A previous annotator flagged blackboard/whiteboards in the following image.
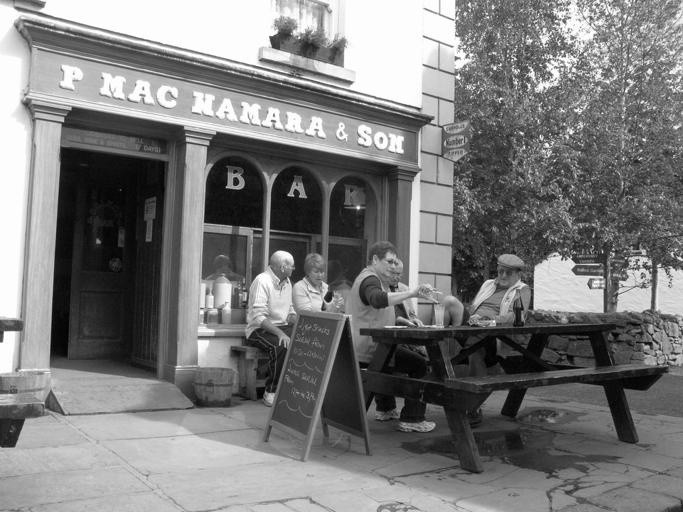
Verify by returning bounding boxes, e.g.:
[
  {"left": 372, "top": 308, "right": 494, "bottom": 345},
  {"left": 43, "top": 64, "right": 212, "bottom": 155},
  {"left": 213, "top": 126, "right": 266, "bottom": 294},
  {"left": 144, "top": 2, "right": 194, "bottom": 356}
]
[{"left": 268, "top": 310, "right": 347, "bottom": 443}]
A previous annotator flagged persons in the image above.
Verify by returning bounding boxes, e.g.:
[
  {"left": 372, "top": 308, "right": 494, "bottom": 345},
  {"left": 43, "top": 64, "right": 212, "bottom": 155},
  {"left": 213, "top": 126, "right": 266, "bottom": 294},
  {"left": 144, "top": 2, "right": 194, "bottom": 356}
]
[
  {"left": 293, "top": 253, "right": 345, "bottom": 314},
  {"left": 245, "top": 250, "right": 297, "bottom": 407},
  {"left": 205, "top": 255, "right": 244, "bottom": 281},
  {"left": 441, "top": 254, "right": 532, "bottom": 428},
  {"left": 344, "top": 241, "right": 442, "bottom": 432}
]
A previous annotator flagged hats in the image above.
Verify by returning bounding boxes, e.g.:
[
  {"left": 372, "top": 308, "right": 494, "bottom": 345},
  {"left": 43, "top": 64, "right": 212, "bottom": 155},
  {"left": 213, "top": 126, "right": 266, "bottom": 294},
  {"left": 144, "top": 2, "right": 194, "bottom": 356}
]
[{"left": 498, "top": 254, "right": 525, "bottom": 271}]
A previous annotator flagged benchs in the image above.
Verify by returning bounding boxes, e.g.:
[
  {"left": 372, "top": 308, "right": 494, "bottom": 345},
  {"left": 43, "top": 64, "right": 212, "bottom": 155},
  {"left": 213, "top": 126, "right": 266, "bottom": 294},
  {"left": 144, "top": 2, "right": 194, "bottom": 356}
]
[{"left": 232, "top": 344, "right": 268, "bottom": 401}]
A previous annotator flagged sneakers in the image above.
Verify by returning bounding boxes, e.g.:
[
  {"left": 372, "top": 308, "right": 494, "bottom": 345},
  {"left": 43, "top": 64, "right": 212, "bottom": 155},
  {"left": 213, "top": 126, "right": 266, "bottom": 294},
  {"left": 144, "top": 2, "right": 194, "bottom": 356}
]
[
  {"left": 374, "top": 409, "right": 400, "bottom": 421},
  {"left": 468, "top": 410, "right": 483, "bottom": 428},
  {"left": 263, "top": 391, "right": 276, "bottom": 407},
  {"left": 396, "top": 420, "right": 436, "bottom": 433}
]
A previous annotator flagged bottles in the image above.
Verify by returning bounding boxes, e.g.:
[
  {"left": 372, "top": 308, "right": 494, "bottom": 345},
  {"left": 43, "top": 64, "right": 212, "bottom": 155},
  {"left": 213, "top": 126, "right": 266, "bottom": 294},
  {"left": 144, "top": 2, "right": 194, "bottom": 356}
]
[
  {"left": 222, "top": 300, "right": 231, "bottom": 326},
  {"left": 206, "top": 287, "right": 215, "bottom": 309},
  {"left": 206, "top": 309, "right": 218, "bottom": 327},
  {"left": 199, "top": 307, "right": 204, "bottom": 325},
  {"left": 214, "top": 272, "right": 232, "bottom": 308},
  {"left": 235, "top": 278, "right": 248, "bottom": 308},
  {"left": 512, "top": 288, "right": 526, "bottom": 328}
]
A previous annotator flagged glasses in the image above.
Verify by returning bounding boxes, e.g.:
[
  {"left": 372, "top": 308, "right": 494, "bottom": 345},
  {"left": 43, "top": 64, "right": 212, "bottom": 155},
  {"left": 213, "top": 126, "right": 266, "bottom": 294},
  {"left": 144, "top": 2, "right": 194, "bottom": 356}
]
[
  {"left": 384, "top": 259, "right": 399, "bottom": 266},
  {"left": 498, "top": 269, "right": 513, "bottom": 276}
]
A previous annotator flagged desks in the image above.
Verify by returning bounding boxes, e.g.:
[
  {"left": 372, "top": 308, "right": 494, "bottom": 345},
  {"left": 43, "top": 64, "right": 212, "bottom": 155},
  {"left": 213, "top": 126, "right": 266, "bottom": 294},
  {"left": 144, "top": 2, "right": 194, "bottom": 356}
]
[{"left": 358, "top": 320, "right": 641, "bottom": 471}]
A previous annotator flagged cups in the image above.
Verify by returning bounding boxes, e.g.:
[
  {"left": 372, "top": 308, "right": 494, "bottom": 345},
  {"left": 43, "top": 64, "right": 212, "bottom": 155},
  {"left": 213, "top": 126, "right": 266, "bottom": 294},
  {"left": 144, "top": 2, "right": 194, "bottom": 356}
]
[
  {"left": 433, "top": 303, "right": 445, "bottom": 327},
  {"left": 418, "top": 289, "right": 443, "bottom": 303}
]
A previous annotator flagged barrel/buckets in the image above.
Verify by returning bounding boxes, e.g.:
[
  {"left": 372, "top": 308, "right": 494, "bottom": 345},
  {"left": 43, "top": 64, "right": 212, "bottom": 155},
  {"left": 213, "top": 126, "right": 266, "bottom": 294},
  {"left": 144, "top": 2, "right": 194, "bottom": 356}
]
[
  {"left": 192, "top": 366, "right": 238, "bottom": 407},
  {"left": 0, "top": 371, "right": 53, "bottom": 408}
]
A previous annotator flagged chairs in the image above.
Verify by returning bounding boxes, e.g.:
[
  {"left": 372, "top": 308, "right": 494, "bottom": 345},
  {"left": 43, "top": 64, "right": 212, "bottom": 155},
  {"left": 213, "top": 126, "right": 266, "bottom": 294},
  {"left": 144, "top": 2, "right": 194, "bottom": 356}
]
[{"left": 441, "top": 353, "right": 669, "bottom": 473}]
